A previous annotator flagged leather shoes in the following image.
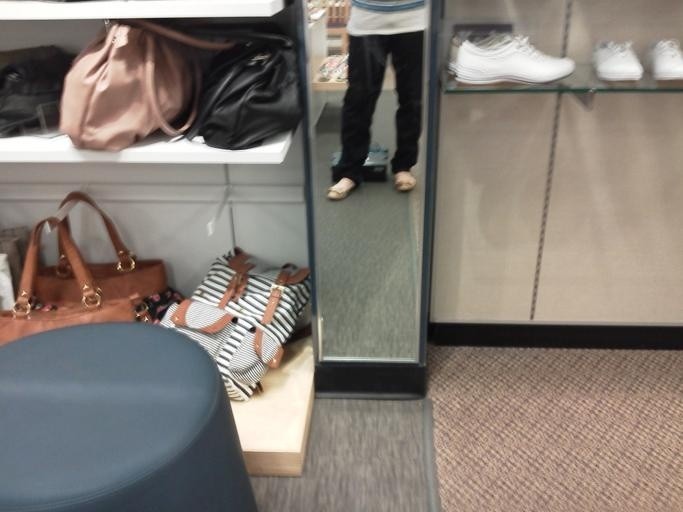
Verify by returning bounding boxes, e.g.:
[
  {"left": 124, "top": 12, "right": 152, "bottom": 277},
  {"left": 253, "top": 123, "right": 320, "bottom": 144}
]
[
  {"left": 328, "top": 186, "right": 355, "bottom": 200},
  {"left": 394, "top": 171, "right": 415, "bottom": 192}
]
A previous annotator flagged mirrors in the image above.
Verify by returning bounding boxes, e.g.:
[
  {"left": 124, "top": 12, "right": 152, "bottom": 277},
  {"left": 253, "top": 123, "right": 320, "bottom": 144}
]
[{"left": 297, "top": 0, "right": 440, "bottom": 400}]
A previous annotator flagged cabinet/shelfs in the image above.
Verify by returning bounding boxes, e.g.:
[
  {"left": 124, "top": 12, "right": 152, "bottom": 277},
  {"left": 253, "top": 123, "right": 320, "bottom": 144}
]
[
  {"left": 430, "top": 2, "right": 683, "bottom": 349},
  {"left": 0, "top": 1, "right": 313, "bottom": 476}
]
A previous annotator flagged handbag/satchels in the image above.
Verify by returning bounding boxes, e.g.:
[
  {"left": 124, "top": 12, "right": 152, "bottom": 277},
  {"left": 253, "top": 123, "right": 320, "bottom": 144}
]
[
  {"left": 1, "top": 46, "right": 79, "bottom": 138},
  {"left": 159, "top": 276, "right": 285, "bottom": 401},
  {"left": 0, "top": 217, "right": 151, "bottom": 346},
  {"left": 186, "top": 21, "right": 303, "bottom": 150},
  {"left": 139, "top": 288, "right": 185, "bottom": 325},
  {"left": 1, "top": 254, "right": 16, "bottom": 311},
  {"left": 191, "top": 246, "right": 314, "bottom": 346},
  {"left": 0, "top": 225, "right": 31, "bottom": 294},
  {"left": 58, "top": 18, "right": 237, "bottom": 153},
  {"left": 35, "top": 191, "right": 166, "bottom": 297}
]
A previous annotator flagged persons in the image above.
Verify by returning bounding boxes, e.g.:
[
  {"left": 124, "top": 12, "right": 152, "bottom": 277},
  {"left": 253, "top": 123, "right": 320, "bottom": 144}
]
[{"left": 325, "top": 0, "right": 429, "bottom": 200}]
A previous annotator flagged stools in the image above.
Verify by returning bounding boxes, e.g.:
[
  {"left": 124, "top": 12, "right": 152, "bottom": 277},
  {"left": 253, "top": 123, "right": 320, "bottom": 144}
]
[{"left": 1, "top": 321, "right": 261, "bottom": 511}]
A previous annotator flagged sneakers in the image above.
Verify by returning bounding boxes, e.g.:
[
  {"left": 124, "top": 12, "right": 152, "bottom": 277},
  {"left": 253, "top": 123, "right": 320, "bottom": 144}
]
[
  {"left": 591, "top": 40, "right": 644, "bottom": 82},
  {"left": 457, "top": 41, "right": 574, "bottom": 83},
  {"left": 447, "top": 31, "right": 514, "bottom": 73},
  {"left": 643, "top": 39, "right": 682, "bottom": 80}
]
